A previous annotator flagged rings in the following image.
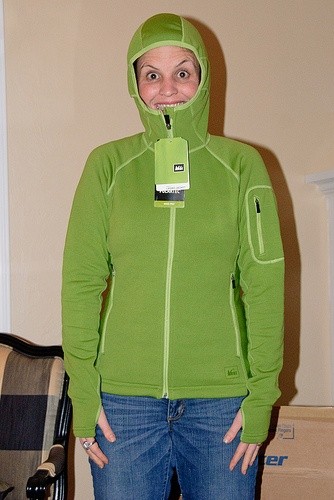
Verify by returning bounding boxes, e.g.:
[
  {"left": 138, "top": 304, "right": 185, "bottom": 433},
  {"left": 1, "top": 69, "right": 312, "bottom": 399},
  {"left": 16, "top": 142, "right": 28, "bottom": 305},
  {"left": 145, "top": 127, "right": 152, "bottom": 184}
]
[{"left": 83, "top": 441, "right": 97, "bottom": 450}]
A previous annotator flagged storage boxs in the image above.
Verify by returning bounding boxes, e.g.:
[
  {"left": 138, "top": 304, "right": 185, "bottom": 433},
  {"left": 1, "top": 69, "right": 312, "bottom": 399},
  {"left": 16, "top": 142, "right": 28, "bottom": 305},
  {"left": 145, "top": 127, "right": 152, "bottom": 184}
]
[{"left": 257, "top": 406, "right": 334, "bottom": 500}]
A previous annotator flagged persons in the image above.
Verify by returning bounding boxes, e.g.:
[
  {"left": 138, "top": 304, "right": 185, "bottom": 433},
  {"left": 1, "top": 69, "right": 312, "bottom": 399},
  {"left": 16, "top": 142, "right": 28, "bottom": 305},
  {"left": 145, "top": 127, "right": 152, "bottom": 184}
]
[{"left": 61, "top": 12, "right": 286, "bottom": 500}]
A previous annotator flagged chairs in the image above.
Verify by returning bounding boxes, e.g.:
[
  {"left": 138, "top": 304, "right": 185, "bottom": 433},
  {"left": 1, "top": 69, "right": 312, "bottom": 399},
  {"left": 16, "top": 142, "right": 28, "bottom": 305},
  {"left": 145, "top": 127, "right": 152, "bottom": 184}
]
[{"left": 0, "top": 333, "right": 76, "bottom": 500}]
